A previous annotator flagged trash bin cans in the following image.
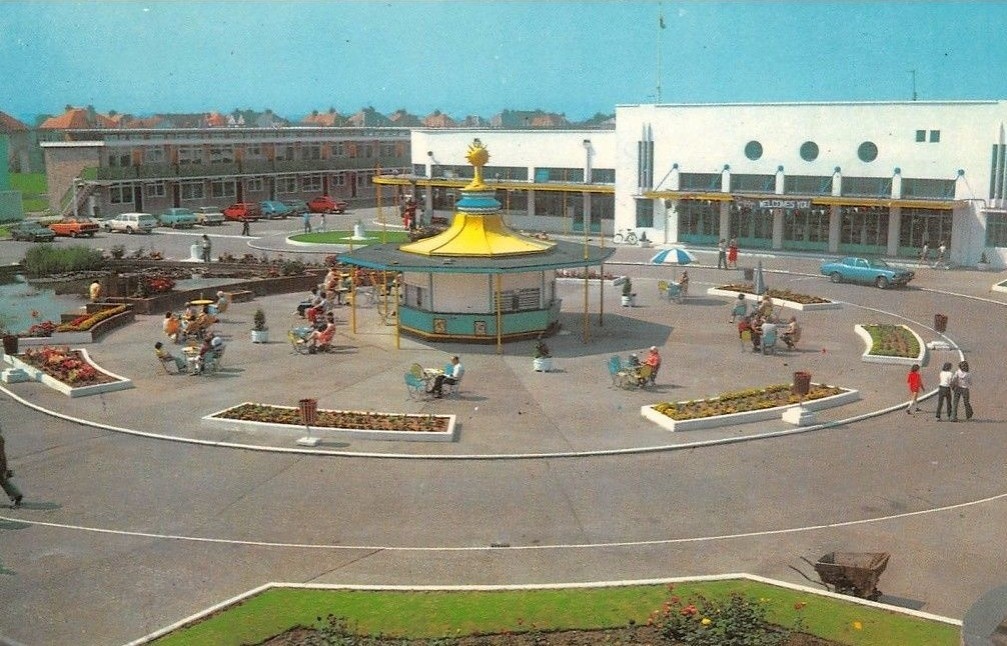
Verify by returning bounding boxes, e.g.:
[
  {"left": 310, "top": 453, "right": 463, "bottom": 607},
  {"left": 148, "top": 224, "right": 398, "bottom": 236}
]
[
  {"left": 933, "top": 313, "right": 947, "bottom": 333},
  {"left": 299, "top": 399, "right": 317, "bottom": 426},
  {"left": 792, "top": 371, "right": 811, "bottom": 396},
  {"left": 744, "top": 267, "right": 754, "bottom": 281},
  {"left": 3, "top": 335, "right": 18, "bottom": 354}
]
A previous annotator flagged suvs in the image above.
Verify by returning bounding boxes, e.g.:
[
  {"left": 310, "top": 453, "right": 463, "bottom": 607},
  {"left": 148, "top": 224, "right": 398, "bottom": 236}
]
[
  {"left": 104, "top": 213, "right": 159, "bottom": 234},
  {"left": 225, "top": 202, "right": 262, "bottom": 222}
]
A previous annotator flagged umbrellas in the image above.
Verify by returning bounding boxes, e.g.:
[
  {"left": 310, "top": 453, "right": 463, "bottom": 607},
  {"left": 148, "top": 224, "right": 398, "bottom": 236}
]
[
  {"left": 754, "top": 263, "right": 766, "bottom": 302},
  {"left": 650, "top": 247, "right": 697, "bottom": 282}
]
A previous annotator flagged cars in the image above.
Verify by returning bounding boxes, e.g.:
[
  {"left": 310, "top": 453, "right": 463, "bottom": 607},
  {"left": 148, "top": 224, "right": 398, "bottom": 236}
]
[
  {"left": 284, "top": 200, "right": 310, "bottom": 217},
  {"left": 820, "top": 255, "right": 916, "bottom": 289},
  {"left": 306, "top": 195, "right": 348, "bottom": 214},
  {"left": 259, "top": 201, "right": 288, "bottom": 220},
  {"left": 193, "top": 206, "right": 226, "bottom": 226},
  {"left": 11, "top": 223, "right": 56, "bottom": 244},
  {"left": 49, "top": 216, "right": 100, "bottom": 238},
  {"left": 157, "top": 207, "right": 199, "bottom": 228}
]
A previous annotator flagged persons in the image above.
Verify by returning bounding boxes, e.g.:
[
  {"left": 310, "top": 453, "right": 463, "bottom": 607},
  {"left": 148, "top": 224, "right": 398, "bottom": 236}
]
[
  {"left": 88, "top": 279, "right": 101, "bottom": 303},
  {"left": 730, "top": 293, "right": 799, "bottom": 350},
  {"left": 642, "top": 345, "right": 661, "bottom": 371},
  {"left": 918, "top": 241, "right": 949, "bottom": 270},
  {"left": 950, "top": 361, "right": 974, "bottom": 421},
  {"left": 678, "top": 270, "right": 689, "bottom": 284},
  {"left": 718, "top": 239, "right": 738, "bottom": 270},
  {"left": 935, "top": 362, "right": 953, "bottom": 421},
  {"left": 906, "top": 364, "right": 925, "bottom": 414},
  {"left": 155, "top": 267, "right": 402, "bottom": 376},
  {"left": 303, "top": 211, "right": 312, "bottom": 234},
  {"left": 0, "top": 436, "right": 23, "bottom": 505},
  {"left": 426, "top": 355, "right": 464, "bottom": 397},
  {"left": 201, "top": 235, "right": 211, "bottom": 262}
]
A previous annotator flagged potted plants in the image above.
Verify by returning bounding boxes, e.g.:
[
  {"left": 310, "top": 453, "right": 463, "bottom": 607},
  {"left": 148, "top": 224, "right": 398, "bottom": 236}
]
[
  {"left": 251, "top": 310, "right": 269, "bottom": 343},
  {"left": 622, "top": 279, "right": 636, "bottom": 307},
  {"left": 534, "top": 338, "right": 553, "bottom": 372}
]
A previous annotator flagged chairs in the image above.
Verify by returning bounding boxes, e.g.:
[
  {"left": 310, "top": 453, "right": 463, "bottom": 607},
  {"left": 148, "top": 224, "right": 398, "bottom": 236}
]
[
  {"left": 608, "top": 355, "right": 651, "bottom": 391},
  {"left": 733, "top": 303, "right": 802, "bottom": 355},
  {"left": 404, "top": 363, "right": 462, "bottom": 402},
  {"left": 160, "top": 343, "right": 226, "bottom": 377},
  {"left": 658, "top": 280, "right": 688, "bottom": 304}
]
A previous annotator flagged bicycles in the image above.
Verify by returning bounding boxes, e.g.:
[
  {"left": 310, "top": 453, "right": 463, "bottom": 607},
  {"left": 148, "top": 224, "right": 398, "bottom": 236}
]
[{"left": 612, "top": 229, "right": 641, "bottom": 245}]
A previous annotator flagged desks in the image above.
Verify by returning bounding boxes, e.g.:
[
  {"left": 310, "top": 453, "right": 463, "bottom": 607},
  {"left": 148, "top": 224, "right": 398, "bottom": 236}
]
[{"left": 191, "top": 299, "right": 214, "bottom": 312}]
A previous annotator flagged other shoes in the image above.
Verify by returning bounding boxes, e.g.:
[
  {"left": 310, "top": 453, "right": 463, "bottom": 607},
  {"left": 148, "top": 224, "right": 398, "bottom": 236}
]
[
  {"left": 915, "top": 408, "right": 922, "bottom": 411},
  {"left": 426, "top": 390, "right": 434, "bottom": 393},
  {"left": 936, "top": 415, "right": 940, "bottom": 419},
  {"left": 968, "top": 411, "right": 973, "bottom": 419},
  {"left": 435, "top": 395, "right": 442, "bottom": 398},
  {"left": 906, "top": 410, "right": 911, "bottom": 414},
  {"left": 16, "top": 496, "right": 22, "bottom": 506}
]
